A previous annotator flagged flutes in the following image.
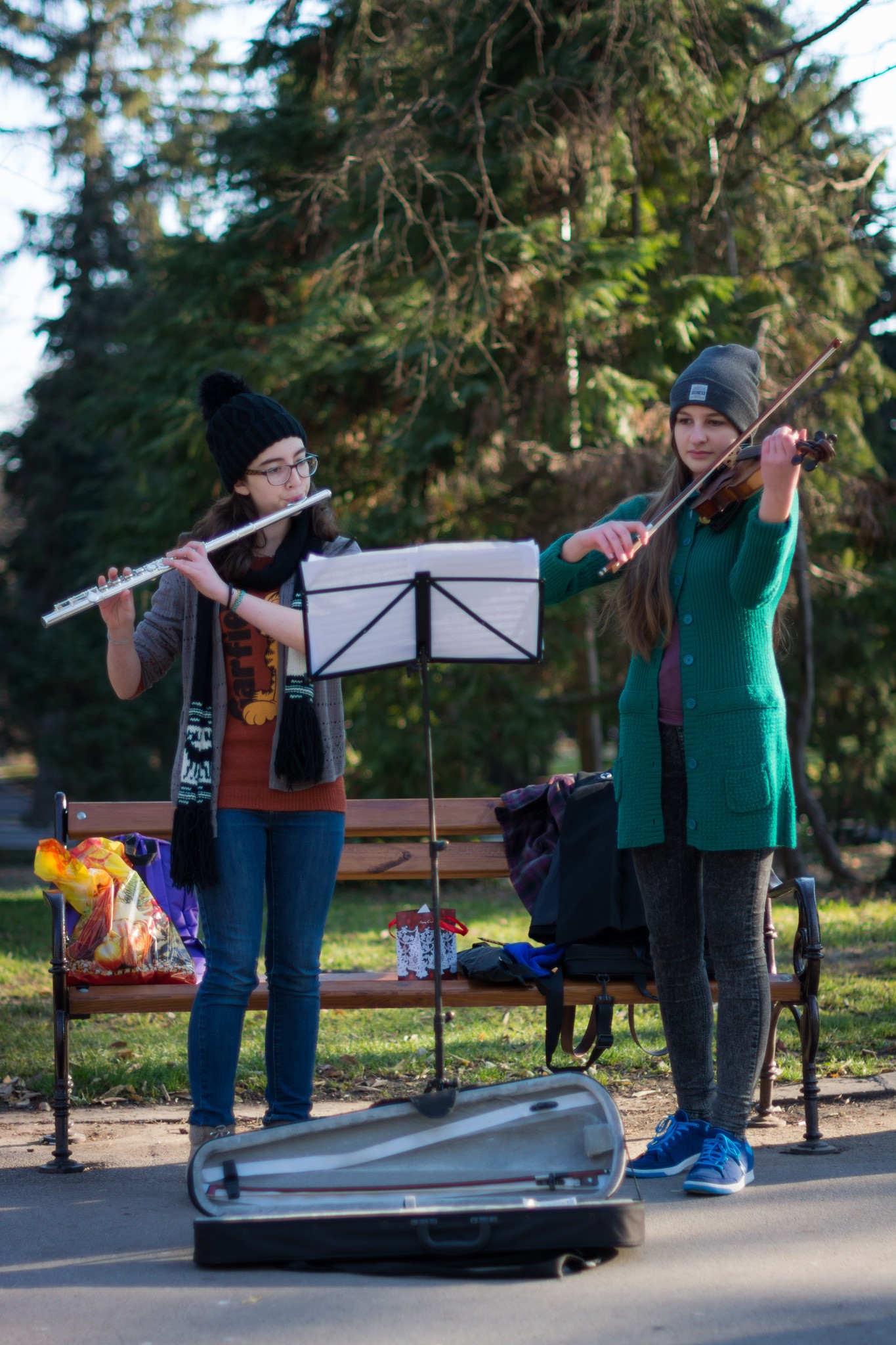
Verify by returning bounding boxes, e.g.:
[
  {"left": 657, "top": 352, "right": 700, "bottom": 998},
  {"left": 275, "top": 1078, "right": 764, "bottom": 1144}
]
[{"left": 38, "top": 488, "right": 332, "bottom": 629}]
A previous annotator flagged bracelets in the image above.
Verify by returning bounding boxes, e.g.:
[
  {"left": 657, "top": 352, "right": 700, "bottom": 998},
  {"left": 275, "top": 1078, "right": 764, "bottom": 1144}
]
[{"left": 224, "top": 582, "right": 246, "bottom": 613}]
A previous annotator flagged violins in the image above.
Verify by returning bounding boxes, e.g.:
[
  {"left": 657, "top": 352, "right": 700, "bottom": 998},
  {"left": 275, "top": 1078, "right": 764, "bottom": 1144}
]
[{"left": 683, "top": 430, "right": 840, "bottom": 524}]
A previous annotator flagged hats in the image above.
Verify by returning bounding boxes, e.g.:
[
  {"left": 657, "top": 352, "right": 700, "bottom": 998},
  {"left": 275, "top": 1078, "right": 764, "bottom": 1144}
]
[
  {"left": 669, "top": 343, "right": 761, "bottom": 441},
  {"left": 196, "top": 369, "right": 307, "bottom": 496}
]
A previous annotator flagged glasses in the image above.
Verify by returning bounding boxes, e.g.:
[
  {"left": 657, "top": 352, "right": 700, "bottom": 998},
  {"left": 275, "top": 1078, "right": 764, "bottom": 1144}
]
[{"left": 245, "top": 452, "right": 319, "bottom": 486}]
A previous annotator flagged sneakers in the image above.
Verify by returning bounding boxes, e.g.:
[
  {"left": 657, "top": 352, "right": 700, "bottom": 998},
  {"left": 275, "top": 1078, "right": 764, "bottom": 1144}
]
[
  {"left": 185, "top": 1124, "right": 235, "bottom": 1183},
  {"left": 683, "top": 1128, "right": 755, "bottom": 1195},
  {"left": 625, "top": 1109, "right": 710, "bottom": 1178}
]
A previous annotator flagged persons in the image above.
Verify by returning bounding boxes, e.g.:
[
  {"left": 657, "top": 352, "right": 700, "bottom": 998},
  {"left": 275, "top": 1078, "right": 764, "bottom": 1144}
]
[
  {"left": 539, "top": 343, "right": 810, "bottom": 1194},
  {"left": 96, "top": 372, "right": 361, "bottom": 1161}
]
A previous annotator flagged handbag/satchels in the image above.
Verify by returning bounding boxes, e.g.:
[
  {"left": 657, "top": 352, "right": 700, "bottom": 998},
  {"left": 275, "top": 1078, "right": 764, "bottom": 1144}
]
[
  {"left": 34, "top": 836, "right": 198, "bottom": 986},
  {"left": 388, "top": 908, "right": 469, "bottom": 981}
]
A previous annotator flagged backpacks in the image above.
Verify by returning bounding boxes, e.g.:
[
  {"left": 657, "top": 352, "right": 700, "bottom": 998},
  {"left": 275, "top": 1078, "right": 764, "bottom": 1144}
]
[
  {"left": 527, "top": 769, "right": 656, "bottom": 985},
  {"left": 65, "top": 833, "right": 207, "bottom": 976}
]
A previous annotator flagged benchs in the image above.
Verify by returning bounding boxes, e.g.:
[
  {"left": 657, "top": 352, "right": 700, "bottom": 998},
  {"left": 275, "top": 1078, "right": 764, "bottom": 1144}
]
[{"left": 39, "top": 790, "right": 840, "bottom": 1172}]
